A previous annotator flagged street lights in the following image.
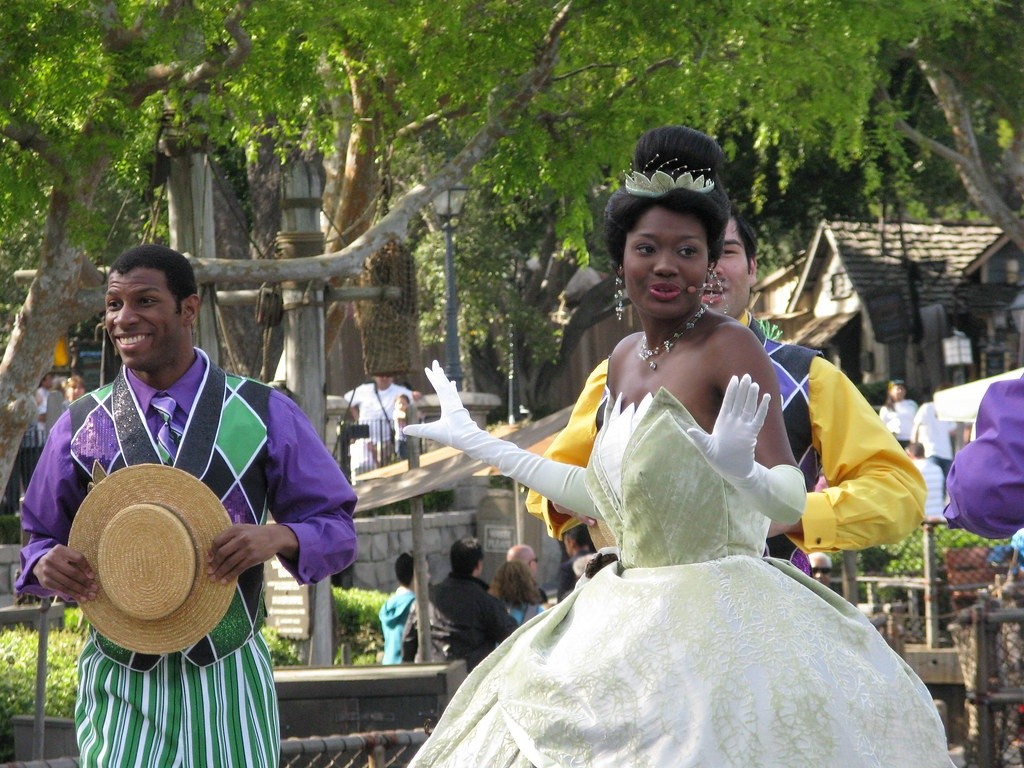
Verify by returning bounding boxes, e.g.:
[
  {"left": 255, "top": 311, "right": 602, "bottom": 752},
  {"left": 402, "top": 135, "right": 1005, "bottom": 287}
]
[
  {"left": 499, "top": 245, "right": 526, "bottom": 425},
  {"left": 433, "top": 181, "right": 469, "bottom": 391},
  {"left": 337, "top": 422, "right": 371, "bottom": 592}
]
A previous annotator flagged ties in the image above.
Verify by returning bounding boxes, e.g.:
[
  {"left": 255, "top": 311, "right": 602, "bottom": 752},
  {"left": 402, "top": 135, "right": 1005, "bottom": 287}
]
[{"left": 150, "top": 395, "right": 183, "bottom": 466}]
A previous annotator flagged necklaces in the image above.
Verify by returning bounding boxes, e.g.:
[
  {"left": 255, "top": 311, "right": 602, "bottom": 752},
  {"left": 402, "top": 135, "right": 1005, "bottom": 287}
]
[{"left": 639, "top": 303, "right": 710, "bottom": 371}]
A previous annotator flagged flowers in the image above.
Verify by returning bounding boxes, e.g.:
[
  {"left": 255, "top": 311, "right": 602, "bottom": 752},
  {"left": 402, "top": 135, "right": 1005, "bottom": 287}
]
[{"left": 621, "top": 160, "right": 716, "bottom": 196}]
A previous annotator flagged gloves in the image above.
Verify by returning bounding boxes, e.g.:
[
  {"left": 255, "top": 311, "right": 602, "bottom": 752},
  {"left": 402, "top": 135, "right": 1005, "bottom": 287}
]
[
  {"left": 687, "top": 370, "right": 809, "bottom": 538},
  {"left": 401, "top": 361, "right": 600, "bottom": 528}
]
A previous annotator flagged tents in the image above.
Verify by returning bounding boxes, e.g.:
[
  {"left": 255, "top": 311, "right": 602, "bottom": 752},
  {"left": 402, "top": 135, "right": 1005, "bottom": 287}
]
[{"left": 932, "top": 368, "right": 1024, "bottom": 443}]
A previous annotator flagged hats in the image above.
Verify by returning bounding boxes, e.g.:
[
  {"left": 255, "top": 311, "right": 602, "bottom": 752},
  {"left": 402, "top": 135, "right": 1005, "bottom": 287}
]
[{"left": 67, "top": 464, "right": 237, "bottom": 657}]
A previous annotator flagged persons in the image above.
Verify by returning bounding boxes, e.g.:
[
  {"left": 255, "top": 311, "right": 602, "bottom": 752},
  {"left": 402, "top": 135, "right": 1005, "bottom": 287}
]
[
  {"left": 378, "top": 524, "right": 598, "bottom": 665},
  {"left": 10, "top": 244, "right": 360, "bottom": 768},
  {"left": 524, "top": 202, "right": 927, "bottom": 577},
  {"left": 2, "top": 372, "right": 86, "bottom": 515},
  {"left": 878, "top": 367, "right": 1024, "bottom": 600},
  {"left": 338, "top": 364, "right": 423, "bottom": 484},
  {"left": 807, "top": 467, "right": 833, "bottom": 592},
  {"left": 402, "top": 123, "right": 951, "bottom": 768}
]
